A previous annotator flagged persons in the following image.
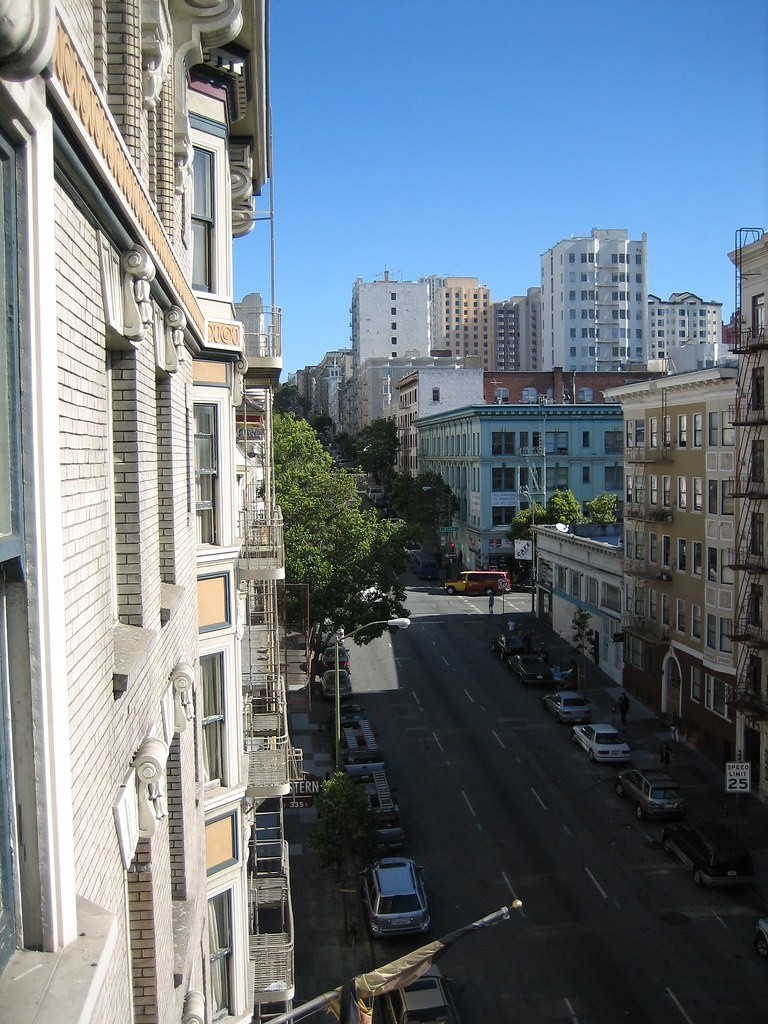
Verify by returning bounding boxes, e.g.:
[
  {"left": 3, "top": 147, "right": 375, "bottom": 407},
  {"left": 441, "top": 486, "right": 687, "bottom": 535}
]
[
  {"left": 489, "top": 593, "right": 494, "bottom": 615},
  {"left": 618, "top": 693, "right": 630, "bottom": 726},
  {"left": 507, "top": 617, "right": 578, "bottom": 678},
  {"left": 659, "top": 743, "right": 671, "bottom": 774},
  {"left": 670, "top": 711, "right": 682, "bottom": 744}
]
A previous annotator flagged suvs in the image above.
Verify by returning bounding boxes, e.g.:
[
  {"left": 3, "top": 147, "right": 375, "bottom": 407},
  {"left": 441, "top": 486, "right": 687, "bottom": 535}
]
[
  {"left": 663, "top": 822, "right": 754, "bottom": 889},
  {"left": 359, "top": 856, "right": 430, "bottom": 940},
  {"left": 615, "top": 769, "right": 688, "bottom": 822}
]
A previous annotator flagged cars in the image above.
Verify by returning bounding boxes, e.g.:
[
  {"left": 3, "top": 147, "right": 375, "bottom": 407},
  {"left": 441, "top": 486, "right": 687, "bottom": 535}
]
[
  {"left": 571, "top": 723, "right": 631, "bottom": 765},
  {"left": 318, "top": 669, "right": 353, "bottom": 701},
  {"left": 505, "top": 655, "right": 554, "bottom": 686},
  {"left": 542, "top": 691, "right": 592, "bottom": 724},
  {"left": 380, "top": 963, "right": 462, "bottom": 1024},
  {"left": 753, "top": 918, "right": 768, "bottom": 959},
  {"left": 329, "top": 704, "right": 365, "bottom": 730},
  {"left": 492, "top": 633, "right": 526, "bottom": 661},
  {"left": 320, "top": 630, "right": 351, "bottom": 676},
  {"left": 317, "top": 432, "right": 399, "bottom": 523},
  {"left": 416, "top": 558, "right": 440, "bottom": 581}
]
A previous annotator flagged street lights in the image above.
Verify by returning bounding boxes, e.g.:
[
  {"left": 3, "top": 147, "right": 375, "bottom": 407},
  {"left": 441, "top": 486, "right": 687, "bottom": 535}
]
[
  {"left": 335, "top": 618, "right": 411, "bottom": 775},
  {"left": 422, "top": 486, "right": 451, "bottom": 580}
]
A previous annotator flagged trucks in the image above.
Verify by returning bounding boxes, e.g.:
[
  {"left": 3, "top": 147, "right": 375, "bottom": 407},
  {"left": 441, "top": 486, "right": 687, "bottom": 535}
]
[{"left": 337, "top": 720, "right": 406, "bottom": 853}]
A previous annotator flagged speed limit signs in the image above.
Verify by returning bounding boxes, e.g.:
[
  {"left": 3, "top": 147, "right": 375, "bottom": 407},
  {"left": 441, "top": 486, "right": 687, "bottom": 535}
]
[{"left": 725, "top": 761, "right": 752, "bottom": 795}]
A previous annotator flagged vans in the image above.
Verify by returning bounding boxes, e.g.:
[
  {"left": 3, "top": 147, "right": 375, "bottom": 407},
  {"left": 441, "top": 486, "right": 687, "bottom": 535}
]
[{"left": 444, "top": 570, "right": 512, "bottom": 595}]
